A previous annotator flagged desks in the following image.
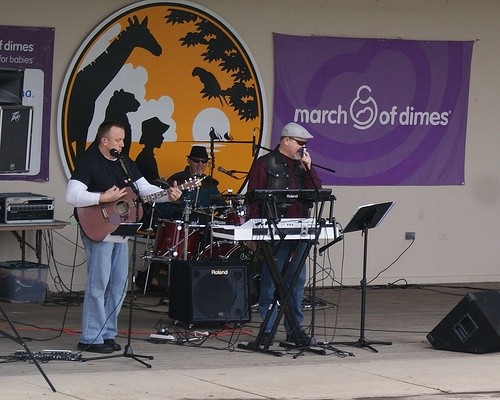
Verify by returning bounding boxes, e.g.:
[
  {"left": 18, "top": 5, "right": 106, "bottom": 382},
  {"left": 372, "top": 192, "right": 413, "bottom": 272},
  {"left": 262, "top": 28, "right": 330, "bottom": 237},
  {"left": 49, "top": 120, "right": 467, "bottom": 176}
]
[{"left": 0, "top": 220, "right": 71, "bottom": 282}]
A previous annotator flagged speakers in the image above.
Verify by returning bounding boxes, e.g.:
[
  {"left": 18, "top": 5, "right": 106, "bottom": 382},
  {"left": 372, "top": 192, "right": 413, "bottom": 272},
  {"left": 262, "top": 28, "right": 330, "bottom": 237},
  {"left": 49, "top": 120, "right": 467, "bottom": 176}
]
[
  {"left": 0, "top": 69, "right": 34, "bottom": 174},
  {"left": 169, "top": 260, "right": 251, "bottom": 324},
  {"left": 425, "top": 291, "right": 500, "bottom": 354}
]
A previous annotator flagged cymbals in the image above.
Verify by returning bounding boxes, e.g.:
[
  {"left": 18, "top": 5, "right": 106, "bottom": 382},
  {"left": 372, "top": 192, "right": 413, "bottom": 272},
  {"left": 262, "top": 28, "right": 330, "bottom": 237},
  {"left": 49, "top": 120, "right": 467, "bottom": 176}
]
[
  {"left": 188, "top": 205, "right": 232, "bottom": 220},
  {"left": 207, "top": 193, "right": 247, "bottom": 200}
]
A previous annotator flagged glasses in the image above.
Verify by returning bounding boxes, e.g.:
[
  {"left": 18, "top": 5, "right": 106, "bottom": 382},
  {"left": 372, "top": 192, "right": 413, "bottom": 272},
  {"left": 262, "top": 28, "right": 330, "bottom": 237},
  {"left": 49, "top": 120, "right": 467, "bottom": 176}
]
[
  {"left": 193, "top": 159, "right": 208, "bottom": 164},
  {"left": 289, "top": 136, "right": 308, "bottom": 146}
]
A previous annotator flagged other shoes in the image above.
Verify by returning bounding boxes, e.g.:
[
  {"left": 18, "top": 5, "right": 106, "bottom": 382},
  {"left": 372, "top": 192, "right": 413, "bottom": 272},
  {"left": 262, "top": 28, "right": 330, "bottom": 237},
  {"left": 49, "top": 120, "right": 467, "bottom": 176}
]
[
  {"left": 286, "top": 332, "right": 315, "bottom": 344},
  {"left": 102, "top": 339, "right": 121, "bottom": 351},
  {"left": 257, "top": 332, "right": 275, "bottom": 346},
  {"left": 77, "top": 341, "right": 114, "bottom": 353}
]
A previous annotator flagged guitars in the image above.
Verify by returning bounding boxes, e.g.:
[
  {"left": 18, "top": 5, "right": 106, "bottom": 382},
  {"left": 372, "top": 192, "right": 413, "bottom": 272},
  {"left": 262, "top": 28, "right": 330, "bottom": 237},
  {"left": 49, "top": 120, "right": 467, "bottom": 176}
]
[{"left": 73, "top": 176, "right": 204, "bottom": 242}]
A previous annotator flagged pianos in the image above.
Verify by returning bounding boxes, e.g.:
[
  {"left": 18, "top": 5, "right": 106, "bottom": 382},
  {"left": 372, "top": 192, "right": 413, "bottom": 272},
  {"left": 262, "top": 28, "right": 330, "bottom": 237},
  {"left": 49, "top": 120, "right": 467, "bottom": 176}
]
[{"left": 208, "top": 217, "right": 339, "bottom": 357}]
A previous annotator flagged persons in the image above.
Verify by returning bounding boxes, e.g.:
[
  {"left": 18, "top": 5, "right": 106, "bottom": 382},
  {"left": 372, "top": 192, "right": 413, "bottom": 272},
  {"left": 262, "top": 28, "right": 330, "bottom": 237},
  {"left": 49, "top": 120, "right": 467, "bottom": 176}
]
[
  {"left": 64, "top": 121, "right": 183, "bottom": 354},
  {"left": 240, "top": 123, "right": 324, "bottom": 347},
  {"left": 161, "top": 146, "right": 232, "bottom": 220}
]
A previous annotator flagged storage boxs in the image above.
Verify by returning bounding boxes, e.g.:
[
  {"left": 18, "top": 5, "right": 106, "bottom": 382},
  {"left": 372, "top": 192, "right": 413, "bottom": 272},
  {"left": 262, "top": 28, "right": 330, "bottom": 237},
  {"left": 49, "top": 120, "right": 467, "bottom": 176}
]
[
  {"left": 0, "top": 261, "right": 49, "bottom": 304},
  {"left": 0, "top": 192, "right": 55, "bottom": 223}
]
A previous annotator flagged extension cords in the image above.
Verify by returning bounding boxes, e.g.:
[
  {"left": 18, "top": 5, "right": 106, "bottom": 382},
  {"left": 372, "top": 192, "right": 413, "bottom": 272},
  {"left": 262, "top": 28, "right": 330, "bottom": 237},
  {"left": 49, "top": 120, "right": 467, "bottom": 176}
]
[{"left": 149, "top": 333, "right": 175, "bottom": 339}]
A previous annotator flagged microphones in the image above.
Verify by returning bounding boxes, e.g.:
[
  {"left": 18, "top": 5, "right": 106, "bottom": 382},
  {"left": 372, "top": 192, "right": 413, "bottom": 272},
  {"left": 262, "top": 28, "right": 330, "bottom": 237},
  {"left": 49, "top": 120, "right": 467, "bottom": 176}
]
[
  {"left": 217, "top": 166, "right": 239, "bottom": 179},
  {"left": 252, "top": 128, "right": 255, "bottom": 156},
  {"left": 110, "top": 149, "right": 126, "bottom": 160}
]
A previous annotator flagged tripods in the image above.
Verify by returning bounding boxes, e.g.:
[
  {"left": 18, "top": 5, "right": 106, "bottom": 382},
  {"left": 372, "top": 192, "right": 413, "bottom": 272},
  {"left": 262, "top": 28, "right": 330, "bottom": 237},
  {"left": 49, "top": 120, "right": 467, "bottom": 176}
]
[
  {"left": 81, "top": 159, "right": 154, "bottom": 367},
  {"left": 288, "top": 201, "right": 396, "bottom": 359}
]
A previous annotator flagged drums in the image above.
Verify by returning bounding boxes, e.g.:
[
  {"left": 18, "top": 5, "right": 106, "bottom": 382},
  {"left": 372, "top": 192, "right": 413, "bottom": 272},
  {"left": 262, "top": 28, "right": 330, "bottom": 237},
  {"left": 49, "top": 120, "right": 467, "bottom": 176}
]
[
  {"left": 224, "top": 205, "right": 247, "bottom": 226},
  {"left": 152, "top": 218, "right": 205, "bottom": 262},
  {"left": 195, "top": 240, "right": 263, "bottom": 308}
]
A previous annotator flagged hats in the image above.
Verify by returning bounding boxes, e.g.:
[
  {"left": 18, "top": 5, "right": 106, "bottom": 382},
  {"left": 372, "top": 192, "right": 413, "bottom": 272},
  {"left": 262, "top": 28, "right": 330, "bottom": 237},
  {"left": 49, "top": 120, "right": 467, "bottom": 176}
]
[
  {"left": 186, "top": 145, "right": 213, "bottom": 161},
  {"left": 280, "top": 122, "right": 314, "bottom": 139}
]
